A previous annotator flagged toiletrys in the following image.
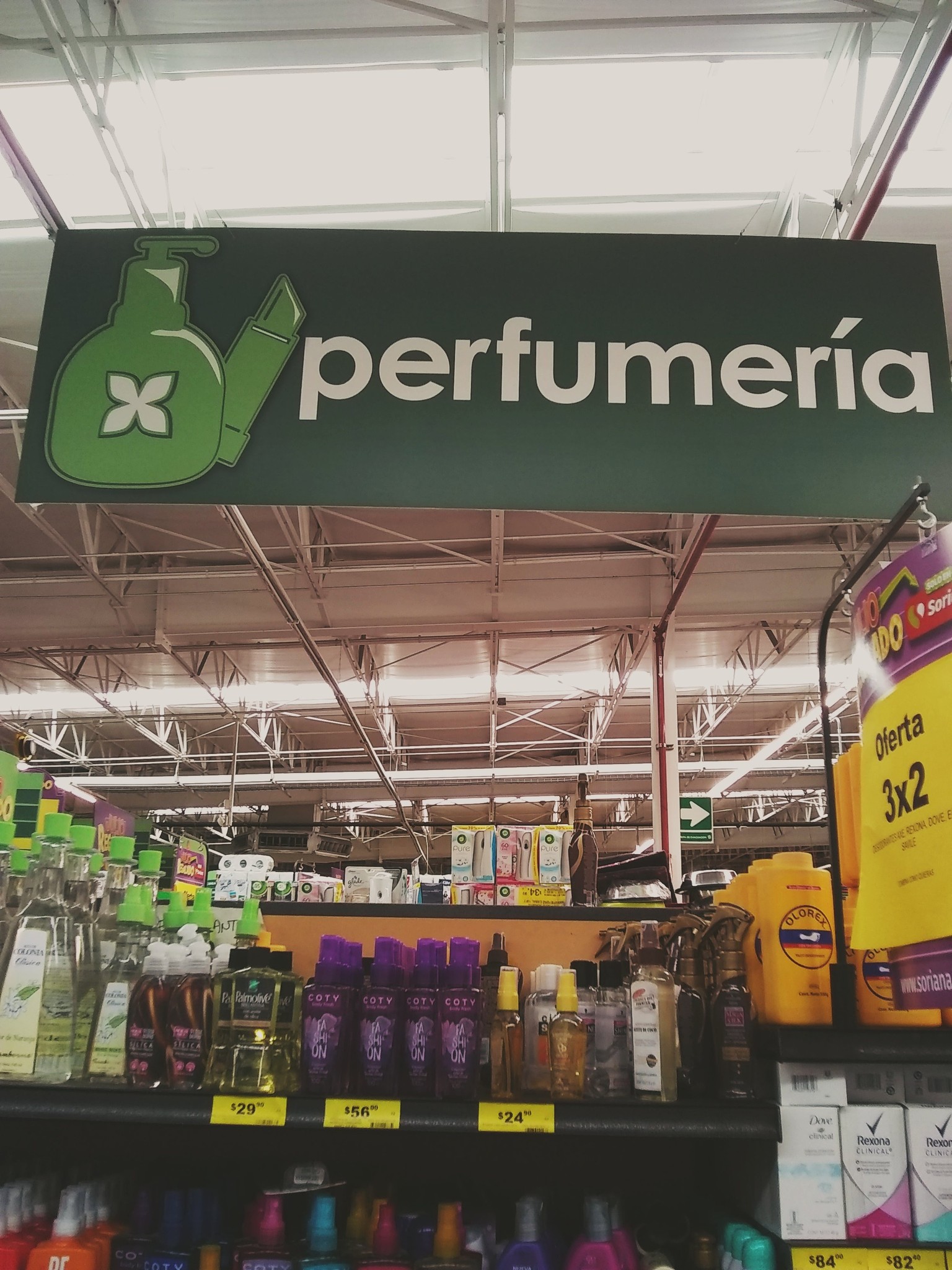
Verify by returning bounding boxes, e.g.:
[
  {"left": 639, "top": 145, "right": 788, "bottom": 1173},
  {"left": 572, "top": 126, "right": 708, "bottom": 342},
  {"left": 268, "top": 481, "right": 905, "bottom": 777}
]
[
  {"left": 1, "top": 806, "right": 948, "bottom": 1101},
  {"left": 0, "top": 1174, "right": 125, "bottom": 1270},
  {"left": 198, "top": 1179, "right": 778, "bottom": 1269}
]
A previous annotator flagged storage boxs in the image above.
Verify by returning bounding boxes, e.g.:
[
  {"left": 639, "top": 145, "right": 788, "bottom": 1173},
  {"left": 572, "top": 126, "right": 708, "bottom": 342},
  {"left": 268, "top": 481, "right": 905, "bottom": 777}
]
[
  {"left": 297, "top": 878, "right": 342, "bottom": 902},
  {"left": 756, "top": 1061, "right": 952, "bottom": 1240},
  {"left": 450, "top": 823, "right": 574, "bottom": 905}
]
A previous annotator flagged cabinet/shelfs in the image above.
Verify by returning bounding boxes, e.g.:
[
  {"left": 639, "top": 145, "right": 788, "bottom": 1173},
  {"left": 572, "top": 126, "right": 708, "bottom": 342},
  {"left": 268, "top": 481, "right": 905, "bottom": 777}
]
[{"left": 755, "top": 1021, "right": 952, "bottom": 1270}]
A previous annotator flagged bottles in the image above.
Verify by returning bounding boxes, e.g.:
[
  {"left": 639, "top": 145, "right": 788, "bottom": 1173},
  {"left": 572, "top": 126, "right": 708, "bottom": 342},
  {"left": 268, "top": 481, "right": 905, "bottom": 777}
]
[
  {"left": 1, "top": 1175, "right": 776, "bottom": 1270},
  {"left": 712, "top": 743, "right": 944, "bottom": 1028},
  {"left": 0, "top": 813, "right": 626, "bottom": 1103}
]
